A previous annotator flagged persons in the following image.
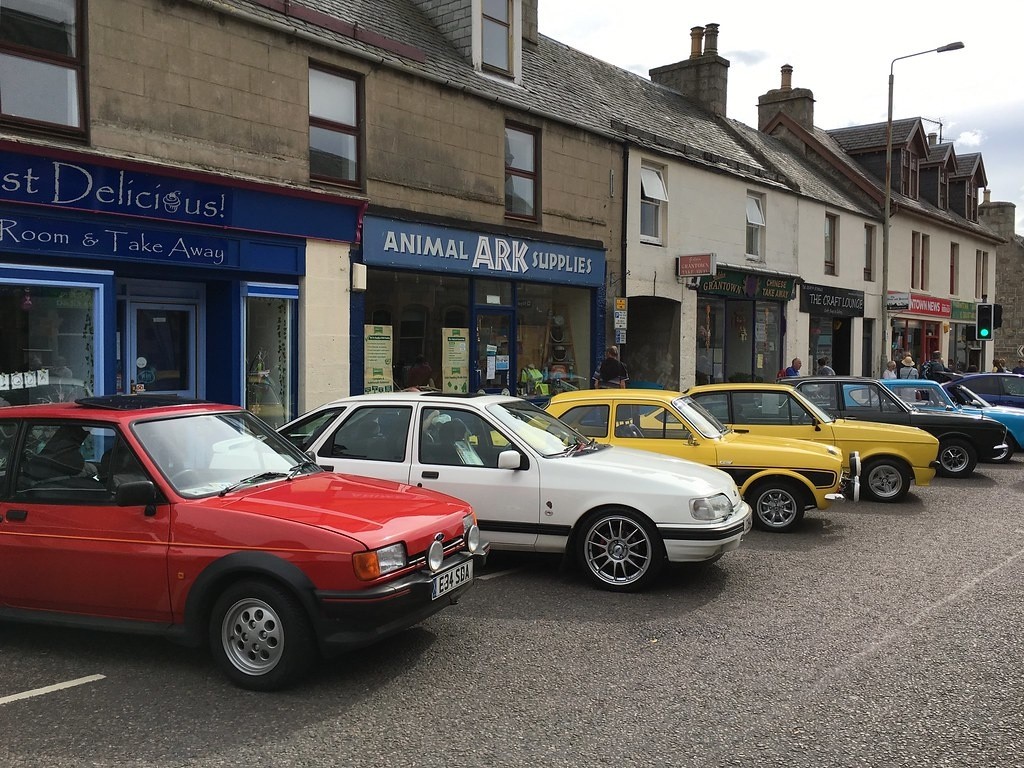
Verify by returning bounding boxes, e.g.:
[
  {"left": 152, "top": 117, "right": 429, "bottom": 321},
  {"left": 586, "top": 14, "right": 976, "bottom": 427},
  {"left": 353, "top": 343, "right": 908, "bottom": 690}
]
[
  {"left": 1012, "top": 359, "right": 1024, "bottom": 374},
  {"left": 408, "top": 355, "right": 437, "bottom": 387},
  {"left": 883, "top": 361, "right": 898, "bottom": 379},
  {"left": 816, "top": 357, "right": 835, "bottom": 376},
  {"left": 777, "top": 358, "right": 802, "bottom": 378},
  {"left": 592, "top": 346, "right": 629, "bottom": 389},
  {"left": 921, "top": 350, "right": 1006, "bottom": 380},
  {"left": 900, "top": 356, "right": 919, "bottom": 379}
]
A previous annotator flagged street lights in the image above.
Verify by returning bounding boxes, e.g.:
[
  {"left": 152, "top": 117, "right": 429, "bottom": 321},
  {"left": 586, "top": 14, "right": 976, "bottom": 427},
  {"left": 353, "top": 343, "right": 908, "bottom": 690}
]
[{"left": 881, "top": 41, "right": 965, "bottom": 379}]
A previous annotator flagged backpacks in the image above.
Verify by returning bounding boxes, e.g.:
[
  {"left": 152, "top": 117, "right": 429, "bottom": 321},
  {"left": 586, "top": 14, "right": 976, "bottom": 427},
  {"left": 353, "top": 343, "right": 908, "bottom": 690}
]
[{"left": 919, "top": 361, "right": 937, "bottom": 379}]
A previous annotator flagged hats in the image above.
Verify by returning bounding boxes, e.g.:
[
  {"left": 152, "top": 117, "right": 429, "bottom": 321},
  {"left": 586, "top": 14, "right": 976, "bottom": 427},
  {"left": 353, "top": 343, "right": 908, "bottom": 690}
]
[
  {"left": 901, "top": 356, "right": 915, "bottom": 366},
  {"left": 932, "top": 351, "right": 942, "bottom": 358}
]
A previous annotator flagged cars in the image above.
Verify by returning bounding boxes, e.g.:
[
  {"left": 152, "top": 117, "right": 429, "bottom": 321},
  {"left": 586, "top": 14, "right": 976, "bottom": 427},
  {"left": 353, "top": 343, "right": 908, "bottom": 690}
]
[
  {"left": 758, "top": 375, "right": 1006, "bottom": 479},
  {"left": 842, "top": 379, "right": 1024, "bottom": 463},
  {"left": 616, "top": 383, "right": 940, "bottom": 502},
  {"left": 209, "top": 390, "right": 757, "bottom": 591},
  {"left": 472, "top": 387, "right": 845, "bottom": 531},
  {"left": 914, "top": 370, "right": 1023, "bottom": 408},
  {"left": 1, "top": 395, "right": 492, "bottom": 691}
]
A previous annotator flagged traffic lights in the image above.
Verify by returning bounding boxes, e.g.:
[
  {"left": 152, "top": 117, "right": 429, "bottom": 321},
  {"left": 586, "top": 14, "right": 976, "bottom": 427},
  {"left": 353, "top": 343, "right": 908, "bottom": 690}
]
[{"left": 976, "top": 303, "right": 994, "bottom": 340}]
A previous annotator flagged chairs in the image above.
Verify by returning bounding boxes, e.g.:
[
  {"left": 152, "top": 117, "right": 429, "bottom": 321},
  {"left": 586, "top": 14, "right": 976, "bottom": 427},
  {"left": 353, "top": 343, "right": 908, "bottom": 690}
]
[
  {"left": 439, "top": 418, "right": 486, "bottom": 466},
  {"left": 108, "top": 444, "right": 156, "bottom": 506},
  {"left": 20, "top": 448, "right": 117, "bottom": 507},
  {"left": 350, "top": 420, "right": 396, "bottom": 460}
]
